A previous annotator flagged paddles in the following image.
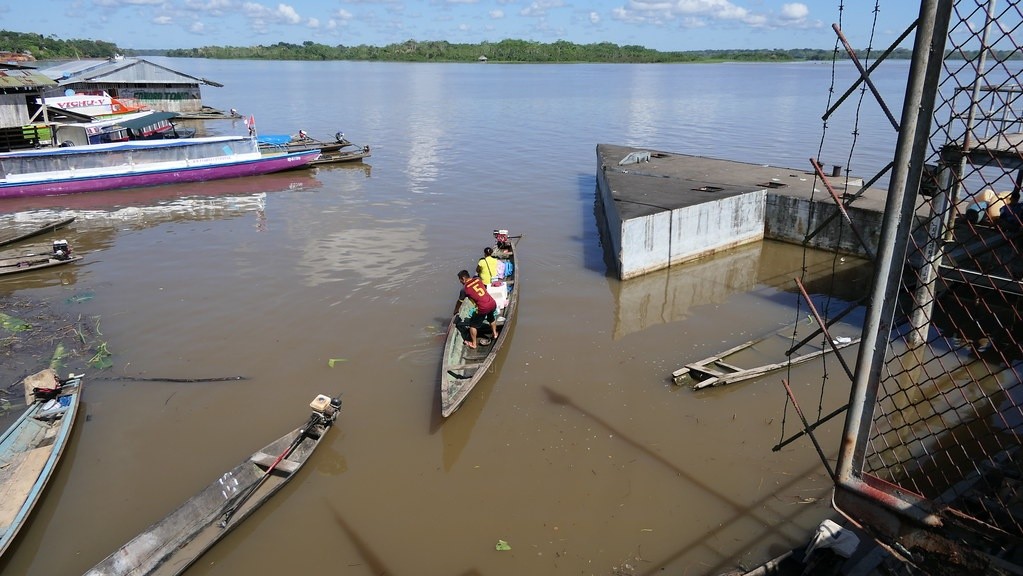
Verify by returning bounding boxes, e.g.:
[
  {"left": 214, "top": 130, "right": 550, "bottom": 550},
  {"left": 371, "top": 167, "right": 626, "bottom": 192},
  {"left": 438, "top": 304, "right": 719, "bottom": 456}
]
[
  {"left": 232, "top": 434, "right": 305, "bottom": 514},
  {"left": 58, "top": 372, "right": 86, "bottom": 382},
  {"left": 218, "top": 416, "right": 318, "bottom": 528},
  {"left": 302, "top": 139, "right": 308, "bottom": 149},
  {"left": 305, "top": 134, "right": 323, "bottom": 143}
]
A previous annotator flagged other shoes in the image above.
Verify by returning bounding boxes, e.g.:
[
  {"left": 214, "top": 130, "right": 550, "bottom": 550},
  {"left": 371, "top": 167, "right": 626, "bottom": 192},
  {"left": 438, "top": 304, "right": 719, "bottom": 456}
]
[{"left": 464, "top": 340, "right": 476, "bottom": 349}]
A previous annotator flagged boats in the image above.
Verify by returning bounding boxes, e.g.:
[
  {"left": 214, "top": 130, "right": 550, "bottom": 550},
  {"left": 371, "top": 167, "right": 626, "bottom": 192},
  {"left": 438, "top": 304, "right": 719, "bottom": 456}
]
[
  {"left": 83, "top": 394, "right": 342, "bottom": 576},
  {"left": 0, "top": 216, "right": 83, "bottom": 275},
  {"left": 0, "top": 369, "right": 86, "bottom": 558},
  {"left": 257, "top": 131, "right": 354, "bottom": 154},
  {"left": 308, "top": 145, "right": 373, "bottom": 165},
  {"left": 54, "top": 110, "right": 196, "bottom": 147},
  {"left": 441, "top": 231, "right": 519, "bottom": 419},
  {"left": 115, "top": 53, "right": 125, "bottom": 60},
  {"left": 0, "top": 135, "right": 320, "bottom": 199}
]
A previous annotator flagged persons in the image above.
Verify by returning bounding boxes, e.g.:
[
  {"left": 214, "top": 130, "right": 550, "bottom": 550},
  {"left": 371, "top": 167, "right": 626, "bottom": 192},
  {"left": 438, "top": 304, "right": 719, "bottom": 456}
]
[
  {"left": 454, "top": 270, "right": 499, "bottom": 349},
  {"left": 299, "top": 130, "right": 304, "bottom": 138},
  {"left": 476, "top": 247, "right": 497, "bottom": 288}
]
